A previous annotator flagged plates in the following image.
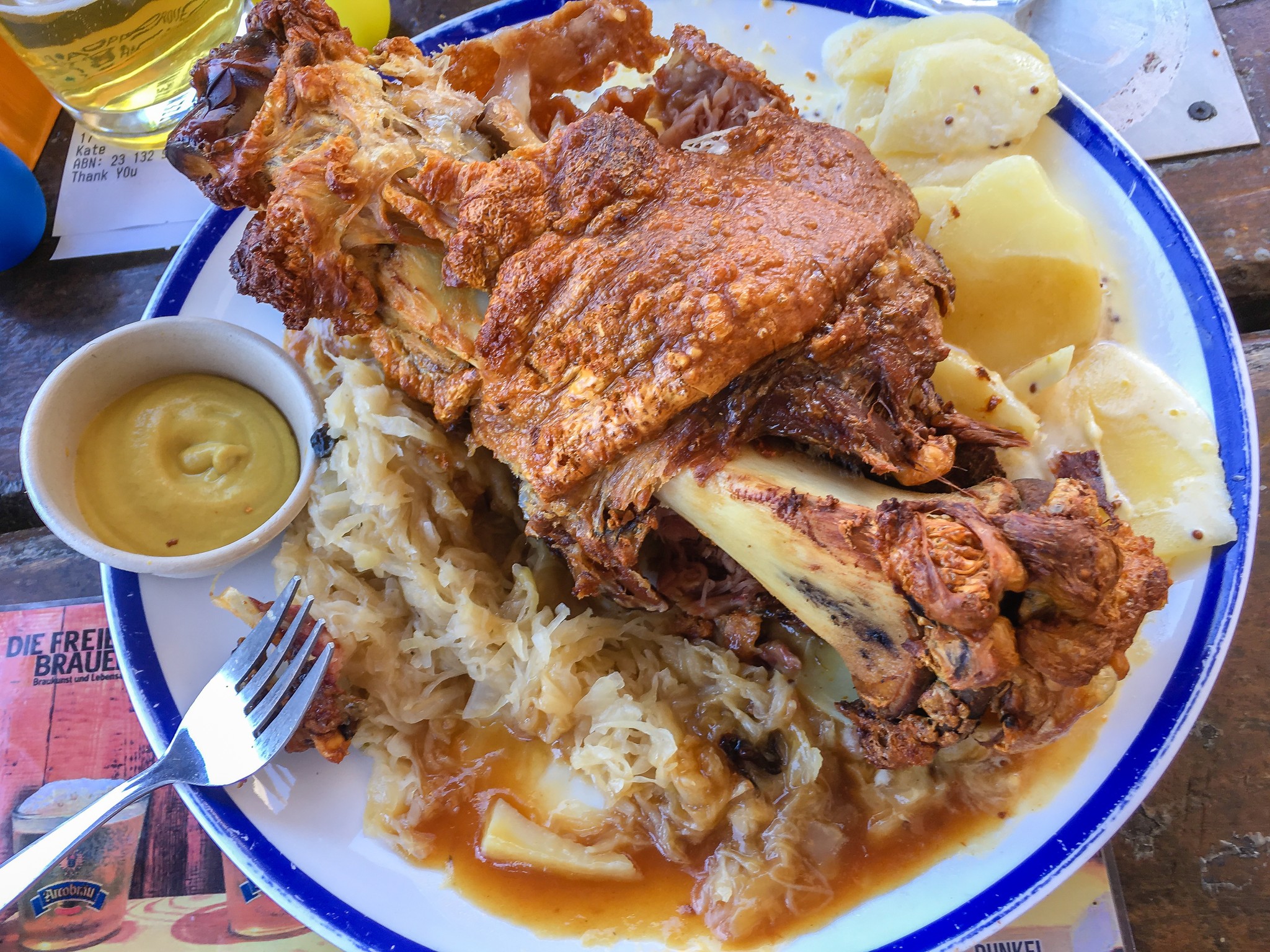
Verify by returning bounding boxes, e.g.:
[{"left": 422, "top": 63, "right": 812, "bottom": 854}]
[{"left": 95, "top": 0, "right": 1263, "bottom": 952}]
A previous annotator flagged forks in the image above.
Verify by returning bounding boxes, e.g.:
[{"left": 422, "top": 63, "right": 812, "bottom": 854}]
[{"left": 0, "top": 571, "right": 336, "bottom": 912}]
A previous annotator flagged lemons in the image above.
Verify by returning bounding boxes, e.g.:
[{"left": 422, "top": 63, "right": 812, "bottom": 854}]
[{"left": 251, "top": 0, "right": 391, "bottom": 59}]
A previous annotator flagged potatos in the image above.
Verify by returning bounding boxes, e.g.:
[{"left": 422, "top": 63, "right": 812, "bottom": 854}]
[{"left": 816, "top": 10, "right": 1237, "bottom": 562}]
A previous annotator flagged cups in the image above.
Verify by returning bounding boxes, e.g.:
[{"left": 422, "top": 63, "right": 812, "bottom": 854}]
[
  {"left": 0, "top": 145, "right": 48, "bottom": 273},
  {"left": 0, "top": 0, "right": 258, "bottom": 151},
  {"left": 19, "top": 316, "right": 325, "bottom": 577}
]
